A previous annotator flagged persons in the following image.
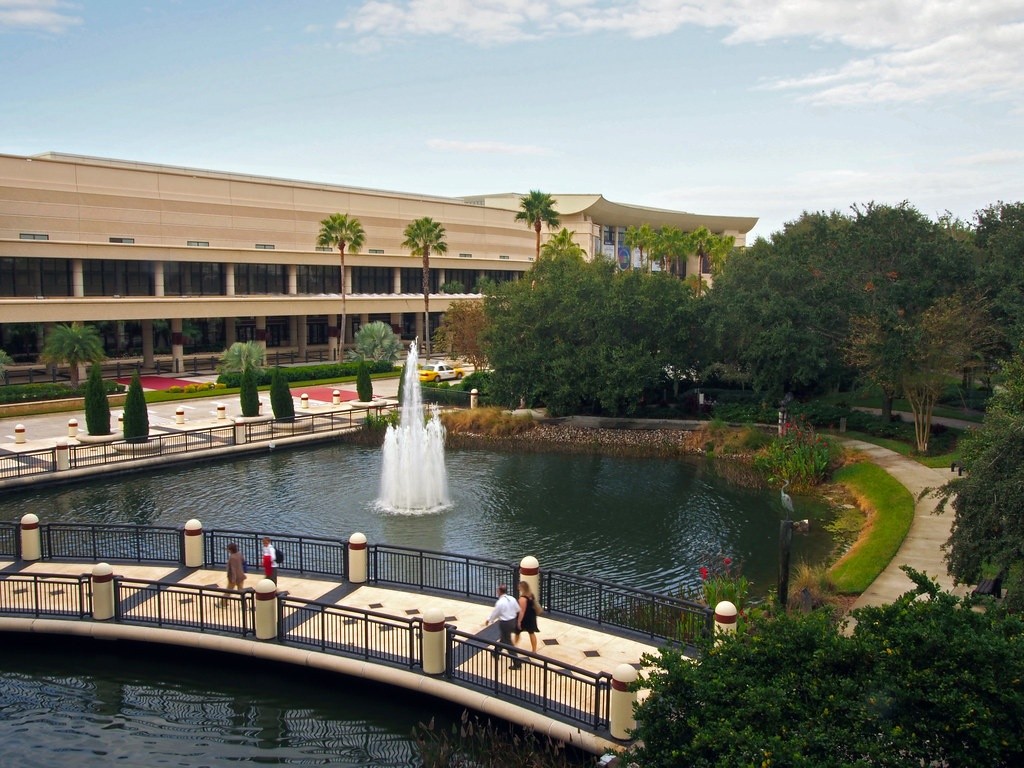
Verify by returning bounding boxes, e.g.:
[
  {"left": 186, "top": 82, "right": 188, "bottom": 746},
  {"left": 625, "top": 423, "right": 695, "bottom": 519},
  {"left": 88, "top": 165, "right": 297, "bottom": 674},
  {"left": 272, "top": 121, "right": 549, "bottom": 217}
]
[
  {"left": 512, "top": 582, "right": 540, "bottom": 652},
  {"left": 216, "top": 543, "right": 249, "bottom": 611},
  {"left": 259, "top": 537, "right": 279, "bottom": 586},
  {"left": 486, "top": 584, "right": 523, "bottom": 670}
]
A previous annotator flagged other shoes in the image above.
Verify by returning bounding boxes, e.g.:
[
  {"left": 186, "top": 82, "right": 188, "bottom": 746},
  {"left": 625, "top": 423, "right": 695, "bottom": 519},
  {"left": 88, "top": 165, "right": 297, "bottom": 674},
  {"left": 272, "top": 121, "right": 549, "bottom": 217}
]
[
  {"left": 491, "top": 651, "right": 499, "bottom": 660},
  {"left": 509, "top": 665, "right": 521, "bottom": 670},
  {"left": 216, "top": 603, "right": 227, "bottom": 608}
]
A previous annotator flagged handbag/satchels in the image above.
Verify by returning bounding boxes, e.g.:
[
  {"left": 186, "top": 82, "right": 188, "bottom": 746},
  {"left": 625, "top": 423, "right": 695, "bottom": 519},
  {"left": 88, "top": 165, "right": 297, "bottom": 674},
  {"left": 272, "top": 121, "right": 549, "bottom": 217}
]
[
  {"left": 243, "top": 561, "right": 248, "bottom": 573},
  {"left": 532, "top": 596, "right": 544, "bottom": 616},
  {"left": 274, "top": 547, "right": 283, "bottom": 563}
]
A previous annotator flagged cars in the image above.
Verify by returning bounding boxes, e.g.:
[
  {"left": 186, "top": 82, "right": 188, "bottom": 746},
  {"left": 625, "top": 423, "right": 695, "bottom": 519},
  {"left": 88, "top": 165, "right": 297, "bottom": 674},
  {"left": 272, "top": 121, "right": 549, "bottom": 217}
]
[{"left": 417, "top": 364, "right": 466, "bottom": 384}]
